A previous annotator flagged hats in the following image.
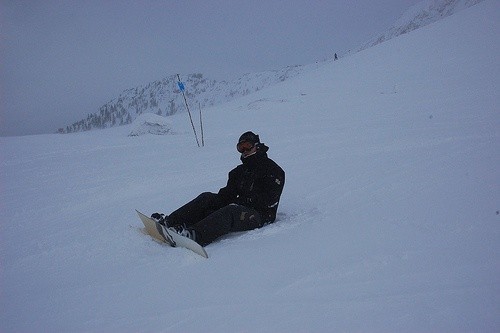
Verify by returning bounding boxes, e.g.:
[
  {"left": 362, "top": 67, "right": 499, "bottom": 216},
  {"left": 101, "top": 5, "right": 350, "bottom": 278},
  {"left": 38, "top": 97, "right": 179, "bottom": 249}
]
[{"left": 238, "top": 131, "right": 260, "bottom": 147}]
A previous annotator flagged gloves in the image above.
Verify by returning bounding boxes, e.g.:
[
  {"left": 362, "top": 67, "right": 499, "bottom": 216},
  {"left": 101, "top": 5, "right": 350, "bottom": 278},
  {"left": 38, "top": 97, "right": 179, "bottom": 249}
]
[
  {"left": 218, "top": 188, "right": 232, "bottom": 201},
  {"left": 235, "top": 191, "right": 256, "bottom": 207}
]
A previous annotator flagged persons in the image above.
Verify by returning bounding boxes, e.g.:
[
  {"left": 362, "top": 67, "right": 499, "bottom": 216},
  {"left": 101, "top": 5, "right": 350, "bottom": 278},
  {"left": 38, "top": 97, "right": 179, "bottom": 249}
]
[{"left": 150, "top": 131, "right": 286, "bottom": 247}]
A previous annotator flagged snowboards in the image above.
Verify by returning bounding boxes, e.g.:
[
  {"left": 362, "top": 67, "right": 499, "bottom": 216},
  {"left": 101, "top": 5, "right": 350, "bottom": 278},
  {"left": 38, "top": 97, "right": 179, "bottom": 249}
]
[{"left": 135, "top": 208, "right": 209, "bottom": 258}]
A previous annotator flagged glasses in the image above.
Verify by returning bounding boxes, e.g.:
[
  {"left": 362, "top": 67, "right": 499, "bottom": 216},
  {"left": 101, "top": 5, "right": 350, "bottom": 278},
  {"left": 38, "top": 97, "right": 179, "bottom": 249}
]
[{"left": 236, "top": 139, "right": 255, "bottom": 153}]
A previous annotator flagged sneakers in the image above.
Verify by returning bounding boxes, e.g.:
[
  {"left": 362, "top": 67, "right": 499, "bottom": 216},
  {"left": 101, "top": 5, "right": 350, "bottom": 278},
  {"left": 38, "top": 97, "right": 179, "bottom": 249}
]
[
  {"left": 151, "top": 212, "right": 170, "bottom": 230},
  {"left": 170, "top": 224, "right": 195, "bottom": 243}
]
